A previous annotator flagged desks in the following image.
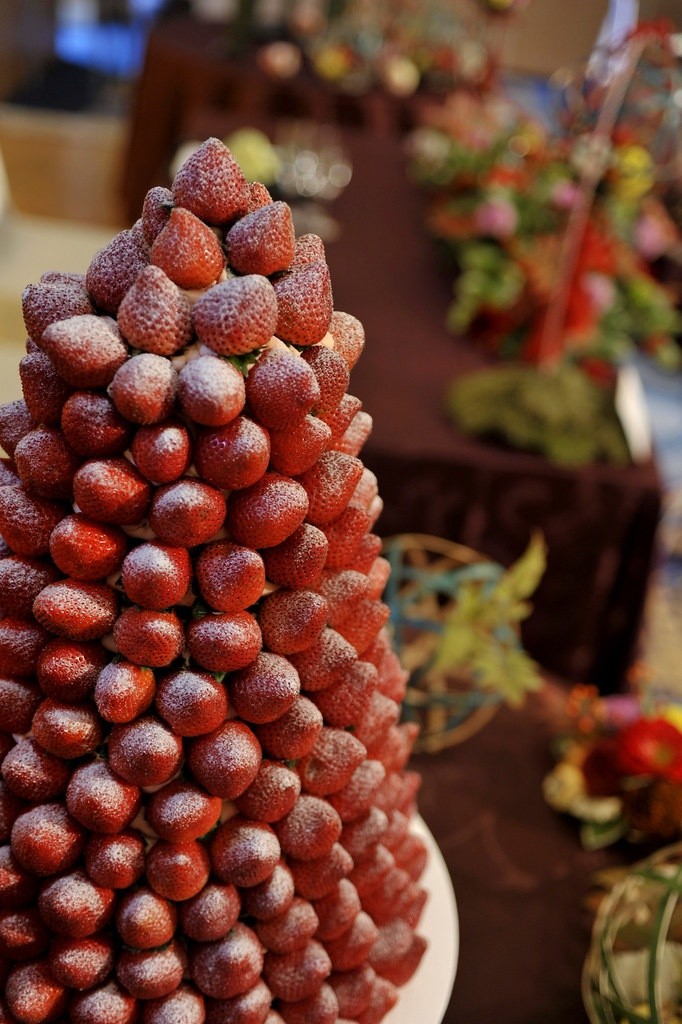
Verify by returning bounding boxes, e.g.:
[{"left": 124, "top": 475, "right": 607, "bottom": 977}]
[
  {"left": 425, "top": 675, "right": 621, "bottom": 1024},
  {"left": 120, "top": 0, "right": 665, "bottom": 689}
]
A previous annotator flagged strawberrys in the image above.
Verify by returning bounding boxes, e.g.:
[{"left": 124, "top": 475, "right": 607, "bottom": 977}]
[{"left": 0, "top": 137, "right": 429, "bottom": 1024}]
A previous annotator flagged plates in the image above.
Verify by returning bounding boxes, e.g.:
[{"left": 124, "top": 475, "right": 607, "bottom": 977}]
[{"left": 382, "top": 802, "right": 459, "bottom": 1024}]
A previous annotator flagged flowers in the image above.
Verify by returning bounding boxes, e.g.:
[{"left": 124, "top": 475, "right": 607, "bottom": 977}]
[{"left": 542, "top": 682, "right": 682, "bottom": 855}]
